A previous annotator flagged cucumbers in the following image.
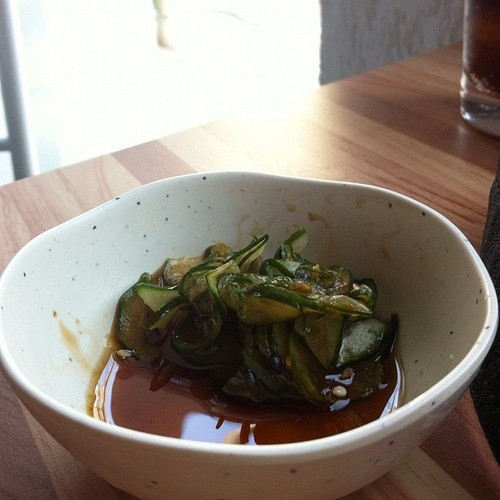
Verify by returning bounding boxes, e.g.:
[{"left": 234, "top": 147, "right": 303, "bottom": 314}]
[{"left": 118, "top": 227, "right": 395, "bottom": 412}]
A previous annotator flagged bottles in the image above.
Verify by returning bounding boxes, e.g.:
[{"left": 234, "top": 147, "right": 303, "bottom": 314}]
[{"left": 461, "top": 0, "right": 500, "bottom": 136}]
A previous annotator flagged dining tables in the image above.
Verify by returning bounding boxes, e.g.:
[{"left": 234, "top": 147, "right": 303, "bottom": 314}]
[{"left": 0, "top": 38, "right": 500, "bottom": 500}]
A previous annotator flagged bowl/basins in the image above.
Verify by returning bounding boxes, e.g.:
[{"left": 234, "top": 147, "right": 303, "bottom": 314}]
[{"left": 0, "top": 171, "right": 498, "bottom": 500}]
